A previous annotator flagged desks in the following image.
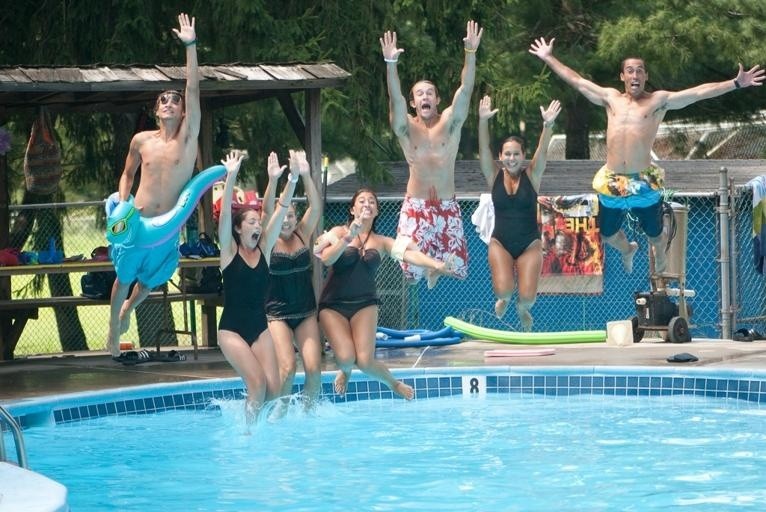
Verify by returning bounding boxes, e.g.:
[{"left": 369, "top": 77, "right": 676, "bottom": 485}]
[{"left": 0, "top": 257, "right": 219, "bottom": 359}]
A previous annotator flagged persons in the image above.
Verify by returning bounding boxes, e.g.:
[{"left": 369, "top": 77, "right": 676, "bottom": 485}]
[
  {"left": 261, "top": 150, "right": 323, "bottom": 402},
  {"left": 478, "top": 95, "right": 562, "bottom": 331},
  {"left": 104, "top": 12, "right": 202, "bottom": 359},
  {"left": 216, "top": 150, "right": 301, "bottom": 424},
  {"left": 529, "top": 36, "right": 765, "bottom": 276},
  {"left": 379, "top": 19, "right": 484, "bottom": 290},
  {"left": 318, "top": 187, "right": 446, "bottom": 402}
]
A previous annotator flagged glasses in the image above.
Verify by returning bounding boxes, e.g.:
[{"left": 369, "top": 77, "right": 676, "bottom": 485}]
[{"left": 161, "top": 95, "right": 180, "bottom": 102}]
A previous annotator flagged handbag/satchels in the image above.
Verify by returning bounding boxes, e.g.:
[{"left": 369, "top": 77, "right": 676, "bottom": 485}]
[
  {"left": 81, "top": 247, "right": 137, "bottom": 299},
  {"left": 178, "top": 233, "right": 223, "bottom": 293}
]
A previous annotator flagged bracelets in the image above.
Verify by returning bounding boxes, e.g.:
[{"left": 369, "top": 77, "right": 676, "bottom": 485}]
[
  {"left": 383, "top": 59, "right": 398, "bottom": 63},
  {"left": 543, "top": 121, "right": 554, "bottom": 130},
  {"left": 278, "top": 202, "right": 289, "bottom": 208},
  {"left": 734, "top": 78, "right": 740, "bottom": 89},
  {"left": 185, "top": 40, "right": 196, "bottom": 47},
  {"left": 464, "top": 48, "right": 477, "bottom": 53},
  {"left": 288, "top": 173, "right": 299, "bottom": 183}
]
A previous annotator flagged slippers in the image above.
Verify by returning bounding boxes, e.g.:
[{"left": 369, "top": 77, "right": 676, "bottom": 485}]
[
  {"left": 667, "top": 353, "right": 698, "bottom": 362},
  {"left": 112, "top": 350, "right": 186, "bottom": 366},
  {"left": 733, "top": 328, "right": 766, "bottom": 342}
]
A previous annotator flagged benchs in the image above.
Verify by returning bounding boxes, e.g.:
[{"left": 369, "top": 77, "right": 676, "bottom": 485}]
[{"left": 0, "top": 291, "right": 219, "bottom": 359}]
